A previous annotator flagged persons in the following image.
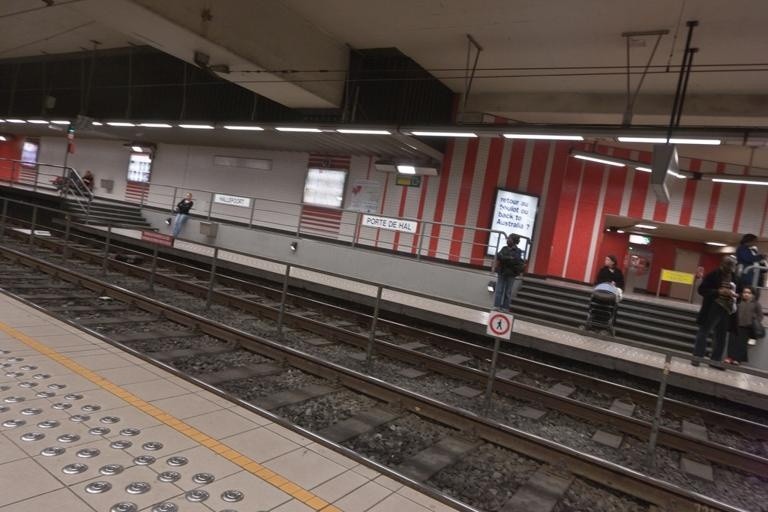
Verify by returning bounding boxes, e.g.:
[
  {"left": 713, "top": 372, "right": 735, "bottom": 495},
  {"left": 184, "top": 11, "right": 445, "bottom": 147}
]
[
  {"left": 690, "top": 255, "right": 738, "bottom": 371},
  {"left": 169, "top": 192, "right": 193, "bottom": 241},
  {"left": 731, "top": 233, "right": 767, "bottom": 300},
  {"left": 596, "top": 255, "right": 625, "bottom": 295},
  {"left": 493, "top": 233, "right": 523, "bottom": 314},
  {"left": 80, "top": 170, "right": 93, "bottom": 195},
  {"left": 724, "top": 286, "right": 762, "bottom": 365}
]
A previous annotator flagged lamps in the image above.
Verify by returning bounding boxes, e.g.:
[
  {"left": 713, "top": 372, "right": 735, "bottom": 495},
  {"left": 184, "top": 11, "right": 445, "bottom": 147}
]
[
  {"left": 701, "top": 170, "right": 768, "bottom": 190},
  {"left": 485, "top": 280, "right": 497, "bottom": 294},
  {"left": 163, "top": 217, "right": 173, "bottom": 226},
  {"left": 289, "top": 240, "right": 301, "bottom": 252},
  {"left": 569, "top": 148, "right": 692, "bottom": 181},
  {"left": 395, "top": 30, "right": 722, "bottom": 152}
]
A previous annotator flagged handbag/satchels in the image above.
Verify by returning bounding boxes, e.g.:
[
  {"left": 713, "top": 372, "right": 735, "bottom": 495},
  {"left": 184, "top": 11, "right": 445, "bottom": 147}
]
[
  {"left": 510, "top": 257, "right": 528, "bottom": 276},
  {"left": 751, "top": 300, "right": 765, "bottom": 340}
]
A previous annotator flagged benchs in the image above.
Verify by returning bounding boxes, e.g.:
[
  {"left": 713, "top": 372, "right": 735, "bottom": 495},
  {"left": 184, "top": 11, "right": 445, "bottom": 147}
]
[{"left": 50, "top": 176, "right": 93, "bottom": 198}]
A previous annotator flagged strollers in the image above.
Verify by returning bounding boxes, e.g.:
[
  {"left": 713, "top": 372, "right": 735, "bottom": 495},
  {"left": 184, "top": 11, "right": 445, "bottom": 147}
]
[{"left": 576, "top": 284, "right": 626, "bottom": 341}]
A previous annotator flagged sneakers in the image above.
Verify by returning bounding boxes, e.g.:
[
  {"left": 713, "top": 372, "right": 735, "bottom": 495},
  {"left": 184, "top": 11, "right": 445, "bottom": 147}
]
[
  {"left": 492, "top": 305, "right": 510, "bottom": 315},
  {"left": 723, "top": 358, "right": 740, "bottom": 366},
  {"left": 707, "top": 363, "right": 728, "bottom": 371},
  {"left": 690, "top": 360, "right": 700, "bottom": 367}
]
